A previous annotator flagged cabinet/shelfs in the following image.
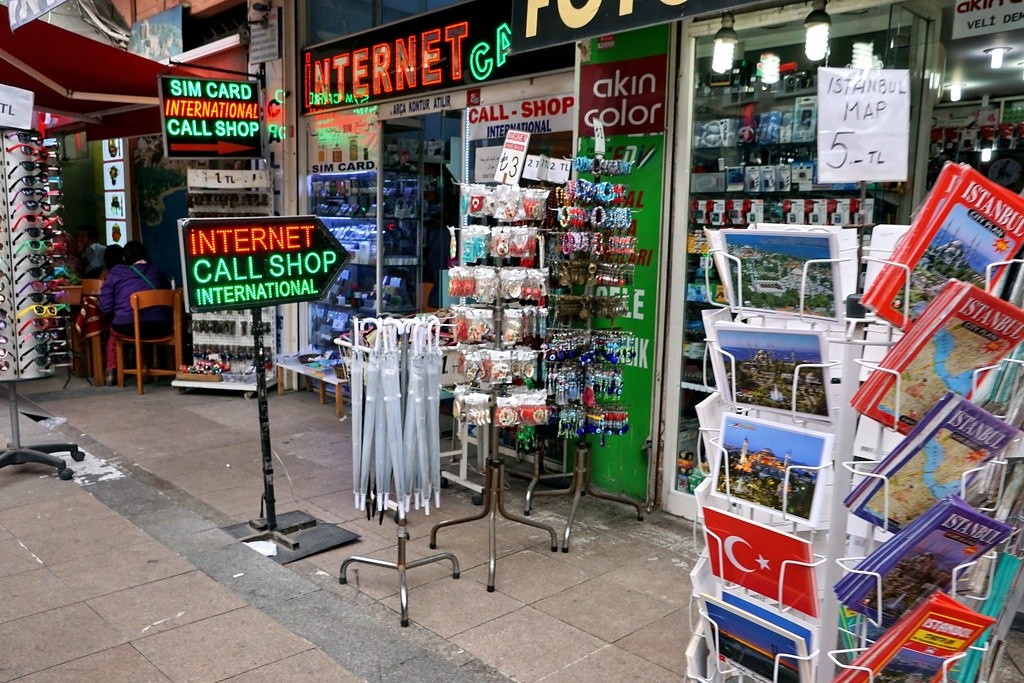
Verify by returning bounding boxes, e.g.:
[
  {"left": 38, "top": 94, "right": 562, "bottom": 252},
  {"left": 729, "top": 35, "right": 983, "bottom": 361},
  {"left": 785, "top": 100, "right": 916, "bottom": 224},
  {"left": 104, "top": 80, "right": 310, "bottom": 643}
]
[
  {"left": 683, "top": 222, "right": 859, "bottom": 683},
  {"left": 858, "top": 223, "right": 1023, "bottom": 683},
  {"left": 305, "top": 116, "right": 445, "bottom": 411}
]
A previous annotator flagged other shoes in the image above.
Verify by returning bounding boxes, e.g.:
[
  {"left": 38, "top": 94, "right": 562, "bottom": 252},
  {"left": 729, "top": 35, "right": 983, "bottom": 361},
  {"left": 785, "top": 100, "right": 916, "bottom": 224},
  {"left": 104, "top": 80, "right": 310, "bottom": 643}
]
[{"left": 104, "top": 375, "right": 115, "bottom": 386}]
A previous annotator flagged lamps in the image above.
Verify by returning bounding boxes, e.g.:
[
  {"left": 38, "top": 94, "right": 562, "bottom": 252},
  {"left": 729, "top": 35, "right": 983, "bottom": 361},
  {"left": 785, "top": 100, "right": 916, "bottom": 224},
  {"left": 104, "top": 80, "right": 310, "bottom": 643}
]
[
  {"left": 944, "top": 63, "right": 966, "bottom": 101},
  {"left": 803, "top": 0, "right": 832, "bottom": 62},
  {"left": 849, "top": 32, "right": 877, "bottom": 80},
  {"left": 983, "top": 33, "right": 1014, "bottom": 69},
  {"left": 759, "top": 47, "right": 781, "bottom": 84},
  {"left": 710, "top": 11, "right": 740, "bottom": 74},
  {"left": 979, "top": 138, "right": 994, "bottom": 162}
]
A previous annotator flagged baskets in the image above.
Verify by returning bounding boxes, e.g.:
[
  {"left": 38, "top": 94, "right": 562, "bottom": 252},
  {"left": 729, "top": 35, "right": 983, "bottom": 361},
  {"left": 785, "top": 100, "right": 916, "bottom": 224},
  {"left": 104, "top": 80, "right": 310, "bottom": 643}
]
[{"left": 334, "top": 358, "right": 348, "bottom": 379}]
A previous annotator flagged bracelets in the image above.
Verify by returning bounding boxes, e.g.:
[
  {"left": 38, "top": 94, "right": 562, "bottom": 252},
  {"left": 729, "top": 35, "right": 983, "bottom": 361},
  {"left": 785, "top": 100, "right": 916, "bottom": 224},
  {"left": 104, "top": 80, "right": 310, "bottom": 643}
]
[{"left": 558, "top": 155, "right": 638, "bottom": 255}]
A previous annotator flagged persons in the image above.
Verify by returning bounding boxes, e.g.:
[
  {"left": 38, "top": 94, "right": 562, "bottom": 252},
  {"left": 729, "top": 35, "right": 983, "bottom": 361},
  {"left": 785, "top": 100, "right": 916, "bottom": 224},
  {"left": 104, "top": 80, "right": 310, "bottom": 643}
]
[
  {"left": 97, "top": 243, "right": 177, "bottom": 387},
  {"left": 74, "top": 224, "right": 104, "bottom": 279},
  {"left": 123, "top": 240, "right": 146, "bottom": 266}
]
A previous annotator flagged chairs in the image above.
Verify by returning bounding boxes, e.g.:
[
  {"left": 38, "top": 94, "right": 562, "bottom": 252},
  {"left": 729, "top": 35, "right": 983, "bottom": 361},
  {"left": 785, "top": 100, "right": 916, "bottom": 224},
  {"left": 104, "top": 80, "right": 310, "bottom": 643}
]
[{"left": 113, "top": 288, "right": 186, "bottom": 394}]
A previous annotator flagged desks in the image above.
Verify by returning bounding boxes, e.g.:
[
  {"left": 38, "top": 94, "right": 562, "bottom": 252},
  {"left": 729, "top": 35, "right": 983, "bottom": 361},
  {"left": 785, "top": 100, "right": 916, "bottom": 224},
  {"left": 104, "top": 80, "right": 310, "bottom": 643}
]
[
  {"left": 50, "top": 277, "right": 106, "bottom": 390},
  {"left": 274, "top": 350, "right": 351, "bottom": 419}
]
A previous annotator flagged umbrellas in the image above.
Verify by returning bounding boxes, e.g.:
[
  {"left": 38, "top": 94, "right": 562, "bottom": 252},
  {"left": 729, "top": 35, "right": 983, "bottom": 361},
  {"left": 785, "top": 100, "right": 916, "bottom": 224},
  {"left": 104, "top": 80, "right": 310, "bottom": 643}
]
[{"left": 347, "top": 316, "right": 445, "bottom": 526}]
